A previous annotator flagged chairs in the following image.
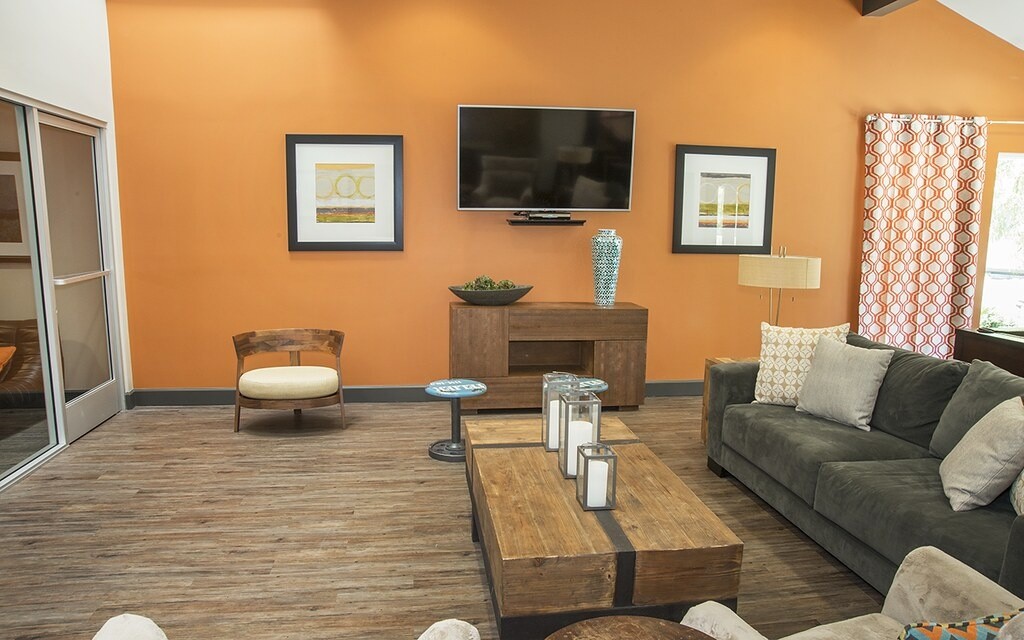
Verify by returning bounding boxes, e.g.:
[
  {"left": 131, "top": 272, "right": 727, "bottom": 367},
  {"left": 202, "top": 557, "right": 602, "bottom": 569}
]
[{"left": 232, "top": 328, "right": 346, "bottom": 433}]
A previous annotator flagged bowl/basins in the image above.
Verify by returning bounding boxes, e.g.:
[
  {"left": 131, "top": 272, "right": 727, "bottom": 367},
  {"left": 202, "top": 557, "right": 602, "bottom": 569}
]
[{"left": 448, "top": 284, "right": 534, "bottom": 306}]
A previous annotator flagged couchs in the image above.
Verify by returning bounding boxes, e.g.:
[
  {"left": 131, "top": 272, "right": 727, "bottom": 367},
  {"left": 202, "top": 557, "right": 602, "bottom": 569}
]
[
  {"left": 680, "top": 545, "right": 1024, "bottom": 640},
  {"left": 708, "top": 328, "right": 1024, "bottom": 597}
]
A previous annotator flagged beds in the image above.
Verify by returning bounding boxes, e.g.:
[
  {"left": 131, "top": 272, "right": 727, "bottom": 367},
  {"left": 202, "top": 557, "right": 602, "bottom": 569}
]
[{"left": 0, "top": 320, "right": 66, "bottom": 441}]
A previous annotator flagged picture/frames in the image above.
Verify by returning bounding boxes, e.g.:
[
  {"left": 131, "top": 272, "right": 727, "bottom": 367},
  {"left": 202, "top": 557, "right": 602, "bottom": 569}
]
[
  {"left": 0, "top": 151, "right": 35, "bottom": 263},
  {"left": 286, "top": 133, "right": 403, "bottom": 252},
  {"left": 672, "top": 142, "right": 777, "bottom": 256}
]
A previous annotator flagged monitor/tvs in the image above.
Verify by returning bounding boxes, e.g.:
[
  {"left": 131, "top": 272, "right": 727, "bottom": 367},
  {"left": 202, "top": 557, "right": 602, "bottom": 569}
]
[{"left": 456, "top": 104, "right": 636, "bottom": 212}]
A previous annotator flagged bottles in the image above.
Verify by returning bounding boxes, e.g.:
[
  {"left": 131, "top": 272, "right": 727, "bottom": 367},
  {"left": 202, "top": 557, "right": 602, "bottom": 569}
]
[{"left": 591, "top": 228, "right": 623, "bottom": 306}]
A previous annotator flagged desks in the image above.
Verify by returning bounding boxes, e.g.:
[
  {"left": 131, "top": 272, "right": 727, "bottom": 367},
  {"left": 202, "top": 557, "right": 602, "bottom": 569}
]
[
  {"left": 545, "top": 616, "right": 716, "bottom": 640},
  {"left": 463, "top": 416, "right": 744, "bottom": 640},
  {"left": 953, "top": 328, "right": 1024, "bottom": 379}
]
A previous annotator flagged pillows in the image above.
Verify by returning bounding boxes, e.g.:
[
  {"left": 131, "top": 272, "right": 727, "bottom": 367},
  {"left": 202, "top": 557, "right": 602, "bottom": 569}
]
[
  {"left": 755, "top": 322, "right": 895, "bottom": 430},
  {"left": 938, "top": 395, "right": 1024, "bottom": 518},
  {"left": 895, "top": 604, "right": 1024, "bottom": 640}
]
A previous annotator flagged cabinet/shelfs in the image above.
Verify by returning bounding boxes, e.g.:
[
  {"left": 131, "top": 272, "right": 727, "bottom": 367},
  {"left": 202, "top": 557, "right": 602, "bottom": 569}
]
[{"left": 448, "top": 302, "right": 649, "bottom": 415}]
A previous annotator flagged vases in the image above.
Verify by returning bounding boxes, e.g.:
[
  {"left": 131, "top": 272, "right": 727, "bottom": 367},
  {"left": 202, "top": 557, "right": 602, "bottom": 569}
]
[{"left": 590, "top": 229, "right": 624, "bottom": 307}]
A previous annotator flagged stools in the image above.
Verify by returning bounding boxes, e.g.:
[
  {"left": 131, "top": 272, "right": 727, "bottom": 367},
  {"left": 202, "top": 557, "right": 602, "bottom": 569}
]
[{"left": 425, "top": 378, "right": 488, "bottom": 462}]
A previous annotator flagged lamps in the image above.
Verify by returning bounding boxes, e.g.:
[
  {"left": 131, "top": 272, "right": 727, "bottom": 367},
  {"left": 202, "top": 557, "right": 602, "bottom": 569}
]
[{"left": 738, "top": 246, "right": 821, "bottom": 327}]
